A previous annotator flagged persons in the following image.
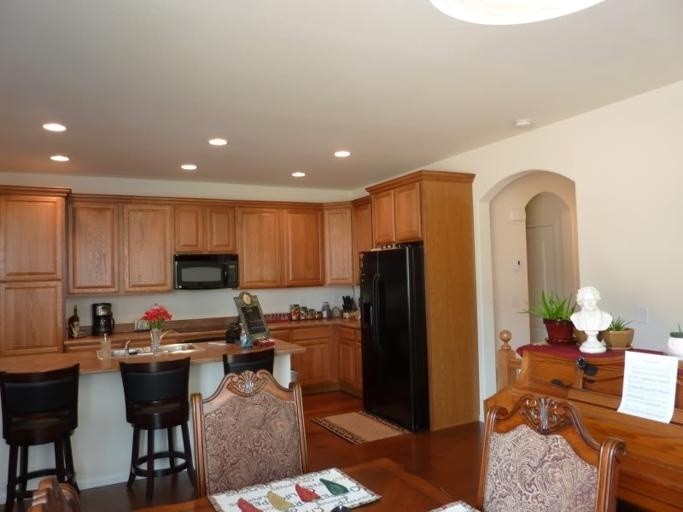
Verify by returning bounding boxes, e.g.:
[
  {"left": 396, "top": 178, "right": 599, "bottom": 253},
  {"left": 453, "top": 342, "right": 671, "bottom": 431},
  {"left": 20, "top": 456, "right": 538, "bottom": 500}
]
[{"left": 570, "top": 286, "right": 613, "bottom": 331}]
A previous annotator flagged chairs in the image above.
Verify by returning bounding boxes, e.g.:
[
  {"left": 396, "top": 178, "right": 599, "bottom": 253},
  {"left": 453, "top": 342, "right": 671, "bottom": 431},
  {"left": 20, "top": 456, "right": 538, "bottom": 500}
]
[
  {"left": 476, "top": 390, "right": 625, "bottom": 512},
  {"left": 190, "top": 369, "right": 307, "bottom": 495},
  {"left": 222, "top": 348, "right": 276, "bottom": 375},
  {"left": 1, "top": 364, "right": 80, "bottom": 511},
  {"left": 120, "top": 358, "right": 199, "bottom": 503},
  {"left": 30, "top": 479, "right": 81, "bottom": 511}
]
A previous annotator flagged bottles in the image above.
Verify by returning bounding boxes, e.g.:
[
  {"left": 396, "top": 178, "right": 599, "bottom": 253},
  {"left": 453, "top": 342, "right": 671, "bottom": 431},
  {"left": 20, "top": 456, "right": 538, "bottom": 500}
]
[
  {"left": 100, "top": 333, "right": 111, "bottom": 364},
  {"left": 69, "top": 305, "right": 80, "bottom": 339},
  {"left": 264, "top": 301, "right": 341, "bottom": 321},
  {"left": 227, "top": 321, "right": 251, "bottom": 349}
]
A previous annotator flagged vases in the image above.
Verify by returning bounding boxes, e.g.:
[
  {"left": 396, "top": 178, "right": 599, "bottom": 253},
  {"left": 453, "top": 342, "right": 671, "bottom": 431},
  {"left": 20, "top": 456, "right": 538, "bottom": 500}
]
[{"left": 149, "top": 319, "right": 165, "bottom": 331}]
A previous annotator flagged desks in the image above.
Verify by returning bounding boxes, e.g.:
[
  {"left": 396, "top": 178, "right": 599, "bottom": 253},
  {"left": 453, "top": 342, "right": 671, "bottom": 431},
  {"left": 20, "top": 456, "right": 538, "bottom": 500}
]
[{"left": 121, "top": 457, "right": 482, "bottom": 512}]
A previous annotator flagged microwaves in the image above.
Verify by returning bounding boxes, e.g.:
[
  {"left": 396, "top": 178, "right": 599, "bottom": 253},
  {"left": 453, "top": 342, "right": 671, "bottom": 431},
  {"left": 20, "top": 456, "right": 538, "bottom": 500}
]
[{"left": 173, "top": 253, "right": 238, "bottom": 289}]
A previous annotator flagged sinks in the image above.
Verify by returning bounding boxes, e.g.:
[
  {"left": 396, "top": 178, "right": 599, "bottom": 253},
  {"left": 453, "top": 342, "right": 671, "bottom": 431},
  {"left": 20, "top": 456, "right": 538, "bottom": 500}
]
[
  {"left": 95, "top": 347, "right": 153, "bottom": 359},
  {"left": 159, "top": 343, "right": 203, "bottom": 354}
]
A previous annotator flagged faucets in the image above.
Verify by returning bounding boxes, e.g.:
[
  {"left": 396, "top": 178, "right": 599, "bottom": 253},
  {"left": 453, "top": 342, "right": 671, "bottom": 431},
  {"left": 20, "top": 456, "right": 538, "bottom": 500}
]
[
  {"left": 159, "top": 329, "right": 175, "bottom": 342},
  {"left": 125, "top": 339, "right": 133, "bottom": 355}
]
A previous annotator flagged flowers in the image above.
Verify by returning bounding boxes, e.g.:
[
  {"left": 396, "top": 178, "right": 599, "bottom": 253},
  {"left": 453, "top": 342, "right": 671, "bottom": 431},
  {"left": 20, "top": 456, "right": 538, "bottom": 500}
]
[{"left": 141, "top": 306, "right": 171, "bottom": 328}]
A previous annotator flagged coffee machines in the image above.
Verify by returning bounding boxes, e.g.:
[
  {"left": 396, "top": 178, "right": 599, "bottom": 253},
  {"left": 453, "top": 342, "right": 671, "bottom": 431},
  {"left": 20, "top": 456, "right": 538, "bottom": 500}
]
[{"left": 92, "top": 302, "right": 114, "bottom": 336}]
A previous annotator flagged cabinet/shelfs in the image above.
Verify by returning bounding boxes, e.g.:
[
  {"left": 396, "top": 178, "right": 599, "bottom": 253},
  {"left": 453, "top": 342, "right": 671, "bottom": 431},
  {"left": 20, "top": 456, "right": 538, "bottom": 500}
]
[
  {"left": 1, "top": 191, "right": 64, "bottom": 355},
  {"left": 324, "top": 199, "right": 368, "bottom": 284},
  {"left": 371, "top": 181, "right": 422, "bottom": 244},
  {"left": 173, "top": 199, "right": 234, "bottom": 254},
  {"left": 336, "top": 327, "right": 363, "bottom": 397},
  {"left": 237, "top": 201, "right": 325, "bottom": 291},
  {"left": 292, "top": 328, "right": 337, "bottom": 395},
  {"left": 67, "top": 196, "right": 174, "bottom": 297}
]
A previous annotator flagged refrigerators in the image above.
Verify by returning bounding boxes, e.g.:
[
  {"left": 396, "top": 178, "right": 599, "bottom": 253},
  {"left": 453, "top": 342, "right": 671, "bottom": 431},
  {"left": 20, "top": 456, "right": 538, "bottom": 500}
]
[{"left": 359, "top": 241, "right": 430, "bottom": 432}]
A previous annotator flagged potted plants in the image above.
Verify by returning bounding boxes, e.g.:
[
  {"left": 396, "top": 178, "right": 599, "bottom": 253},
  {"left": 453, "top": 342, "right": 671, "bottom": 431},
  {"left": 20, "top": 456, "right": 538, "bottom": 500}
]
[
  {"left": 516, "top": 287, "right": 576, "bottom": 345},
  {"left": 603, "top": 315, "right": 636, "bottom": 350},
  {"left": 667, "top": 325, "right": 682, "bottom": 362}
]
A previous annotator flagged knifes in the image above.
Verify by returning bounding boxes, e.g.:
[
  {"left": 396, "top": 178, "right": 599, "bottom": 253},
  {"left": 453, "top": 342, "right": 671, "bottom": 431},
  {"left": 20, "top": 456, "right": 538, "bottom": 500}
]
[{"left": 342, "top": 296, "right": 354, "bottom": 312}]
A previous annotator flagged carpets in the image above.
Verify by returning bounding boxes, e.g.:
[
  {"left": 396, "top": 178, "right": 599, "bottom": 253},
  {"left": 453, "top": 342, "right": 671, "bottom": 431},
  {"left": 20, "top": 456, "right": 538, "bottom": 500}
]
[{"left": 311, "top": 411, "right": 412, "bottom": 444}]
